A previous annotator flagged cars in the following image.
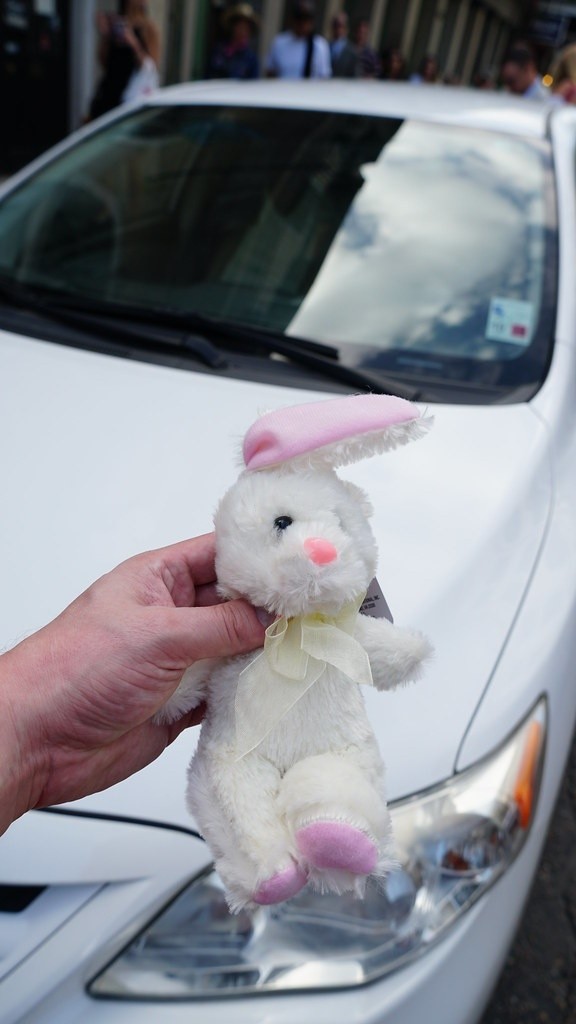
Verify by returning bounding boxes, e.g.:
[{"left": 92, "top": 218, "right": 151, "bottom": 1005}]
[{"left": 0, "top": 78, "right": 576, "bottom": 1024}]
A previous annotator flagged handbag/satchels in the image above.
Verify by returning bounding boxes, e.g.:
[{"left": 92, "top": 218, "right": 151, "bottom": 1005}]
[{"left": 122, "top": 55, "right": 161, "bottom": 105}]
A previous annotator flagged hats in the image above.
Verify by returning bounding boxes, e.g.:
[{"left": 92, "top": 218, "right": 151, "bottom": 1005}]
[{"left": 224, "top": 3, "right": 260, "bottom": 30}]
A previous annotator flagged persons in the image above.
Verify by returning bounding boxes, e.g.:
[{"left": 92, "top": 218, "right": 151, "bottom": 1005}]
[
  {"left": 5, "top": 0, "right": 576, "bottom": 184},
  {"left": 1, "top": 533, "right": 275, "bottom": 834}
]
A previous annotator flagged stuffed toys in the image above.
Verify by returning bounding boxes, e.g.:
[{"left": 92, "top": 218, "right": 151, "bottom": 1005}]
[{"left": 156, "top": 395, "right": 434, "bottom": 914}]
